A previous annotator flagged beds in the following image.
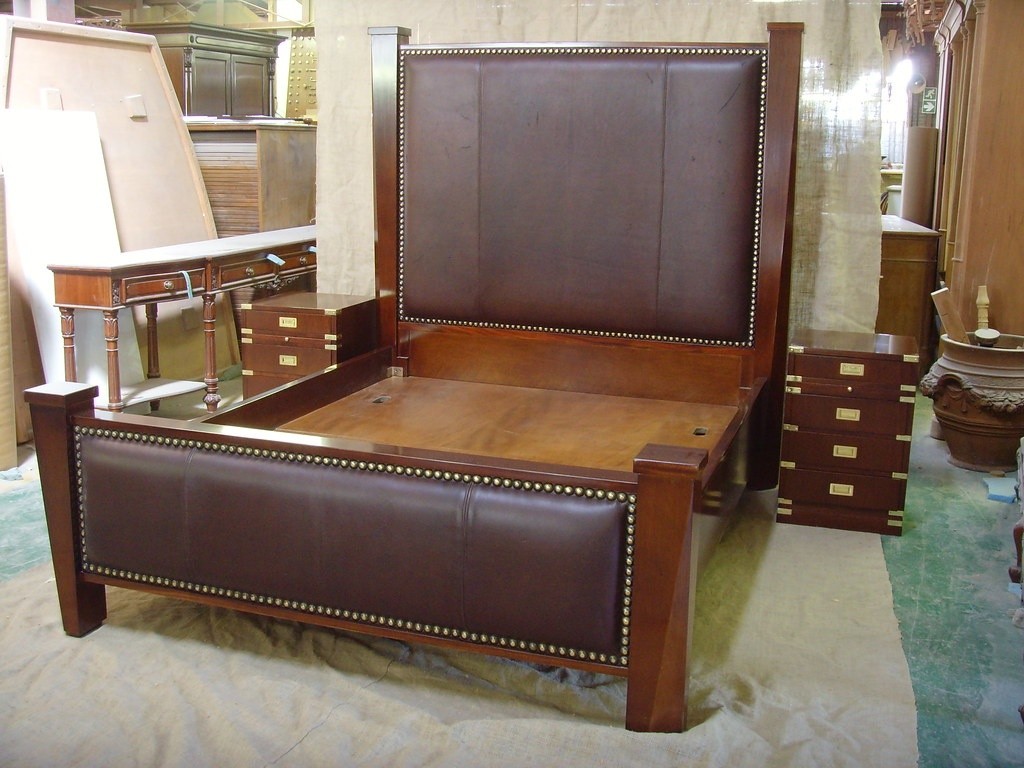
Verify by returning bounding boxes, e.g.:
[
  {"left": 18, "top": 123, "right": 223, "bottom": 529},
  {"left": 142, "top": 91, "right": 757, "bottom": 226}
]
[{"left": 21, "top": 23, "right": 815, "bottom": 734}]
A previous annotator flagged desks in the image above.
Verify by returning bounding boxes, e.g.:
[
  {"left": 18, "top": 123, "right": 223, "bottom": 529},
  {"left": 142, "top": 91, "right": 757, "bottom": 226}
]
[{"left": 45, "top": 223, "right": 319, "bottom": 414}]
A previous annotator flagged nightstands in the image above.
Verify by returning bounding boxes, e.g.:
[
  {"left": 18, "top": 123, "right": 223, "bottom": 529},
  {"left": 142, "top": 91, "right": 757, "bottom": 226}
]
[
  {"left": 776, "top": 325, "right": 919, "bottom": 536},
  {"left": 240, "top": 293, "right": 374, "bottom": 402}
]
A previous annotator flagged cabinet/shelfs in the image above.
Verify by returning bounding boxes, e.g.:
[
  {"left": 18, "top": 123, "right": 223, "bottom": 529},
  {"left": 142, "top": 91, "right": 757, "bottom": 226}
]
[{"left": 879, "top": 215, "right": 941, "bottom": 381}]
